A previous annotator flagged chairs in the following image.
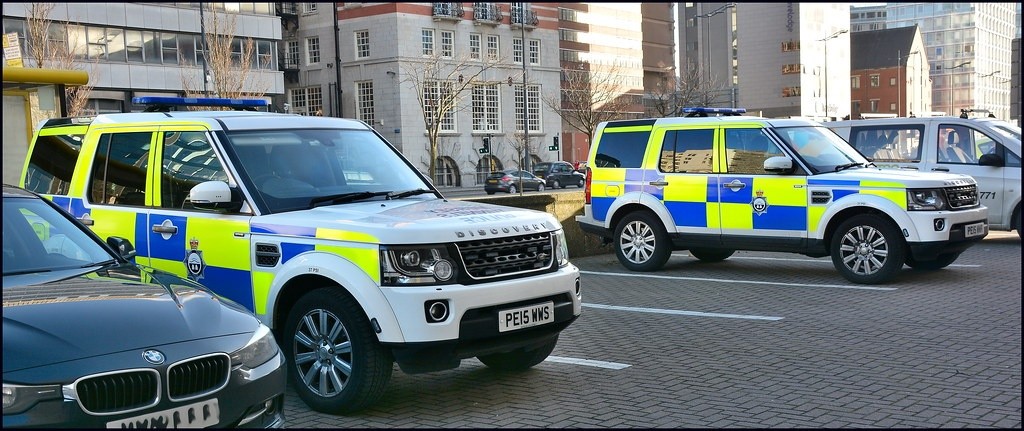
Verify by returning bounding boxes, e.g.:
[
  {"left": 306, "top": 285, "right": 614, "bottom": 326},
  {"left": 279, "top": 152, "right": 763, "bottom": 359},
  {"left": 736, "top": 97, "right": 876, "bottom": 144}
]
[
  {"left": 864, "top": 130, "right": 902, "bottom": 159},
  {"left": 947, "top": 130, "right": 975, "bottom": 163}
]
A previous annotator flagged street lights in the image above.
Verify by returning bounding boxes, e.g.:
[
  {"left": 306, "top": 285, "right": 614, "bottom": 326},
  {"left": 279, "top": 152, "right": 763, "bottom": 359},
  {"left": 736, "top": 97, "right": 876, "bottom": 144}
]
[
  {"left": 944, "top": 61, "right": 973, "bottom": 115},
  {"left": 897, "top": 50, "right": 920, "bottom": 119},
  {"left": 698, "top": 3, "right": 736, "bottom": 99},
  {"left": 820, "top": 28, "right": 850, "bottom": 116}
]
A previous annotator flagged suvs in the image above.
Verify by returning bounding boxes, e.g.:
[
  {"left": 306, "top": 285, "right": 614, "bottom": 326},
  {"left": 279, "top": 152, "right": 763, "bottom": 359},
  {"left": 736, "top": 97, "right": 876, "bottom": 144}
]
[
  {"left": 575, "top": 107, "right": 991, "bottom": 285},
  {"left": 16, "top": 95, "right": 582, "bottom": 417},
  {"left": 531, "top": 160, "right": 585, "bottom": 190}
]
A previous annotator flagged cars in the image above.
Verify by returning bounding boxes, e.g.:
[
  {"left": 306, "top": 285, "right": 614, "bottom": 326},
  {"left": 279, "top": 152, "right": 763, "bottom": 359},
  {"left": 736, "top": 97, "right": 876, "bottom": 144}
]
[
  {"left": 4, "top": 183, "right": 290, "bottom": 431},
  {"left": 484, "top": 169, "right": 547, "bottom": 195}
]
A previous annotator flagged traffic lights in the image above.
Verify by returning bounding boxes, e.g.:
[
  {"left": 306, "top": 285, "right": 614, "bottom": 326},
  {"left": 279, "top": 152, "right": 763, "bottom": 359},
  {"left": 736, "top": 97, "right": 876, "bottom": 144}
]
[
  {"left": 479, "top": 136, "right": 489, "bottom": 154},
  {"left": 549, "top": 137, "right": 560, "bottom": 151}
]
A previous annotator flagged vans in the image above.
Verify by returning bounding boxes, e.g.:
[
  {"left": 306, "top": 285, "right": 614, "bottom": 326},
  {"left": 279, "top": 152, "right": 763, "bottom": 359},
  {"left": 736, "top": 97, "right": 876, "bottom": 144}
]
[{"left": 798, "top": 116, "right": 1022, "bottom": 242}]
